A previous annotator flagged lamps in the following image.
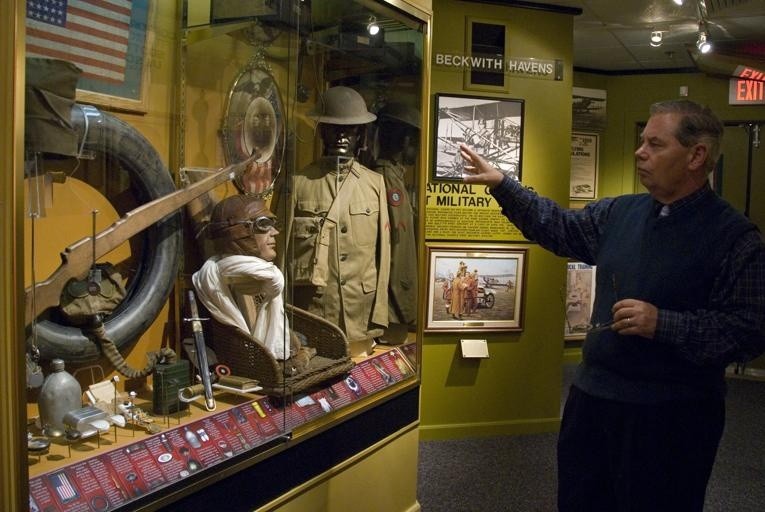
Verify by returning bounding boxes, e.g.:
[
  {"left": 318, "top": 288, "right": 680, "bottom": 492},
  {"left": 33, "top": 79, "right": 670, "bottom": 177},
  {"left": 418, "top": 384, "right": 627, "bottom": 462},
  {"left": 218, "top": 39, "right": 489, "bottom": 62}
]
[
  {"left": 649, "top": 29, "right": 664, "bottom": 49},
  {"left": 695, "top": 21, "right": 712, "bottom": 56},
  {"left": 366, "top": 13, "right": 381, "bottom": 37}
]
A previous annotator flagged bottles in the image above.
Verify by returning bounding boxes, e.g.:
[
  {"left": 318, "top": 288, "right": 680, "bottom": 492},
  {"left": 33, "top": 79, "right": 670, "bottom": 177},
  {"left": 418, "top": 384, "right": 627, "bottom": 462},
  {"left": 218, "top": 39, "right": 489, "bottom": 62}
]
[
  {"left": 154, "top": 348, "right": 192, "bottom": 413},
  {"left": 38, "top": 358, "right": 82, "bottom": 437}
]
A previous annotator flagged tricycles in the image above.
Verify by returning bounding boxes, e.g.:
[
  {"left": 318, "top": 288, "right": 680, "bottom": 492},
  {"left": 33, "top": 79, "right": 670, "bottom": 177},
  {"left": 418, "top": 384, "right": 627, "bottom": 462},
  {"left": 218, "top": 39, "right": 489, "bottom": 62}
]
[{"left": 443, "top": 276, "right": 497, "bottom": 314}]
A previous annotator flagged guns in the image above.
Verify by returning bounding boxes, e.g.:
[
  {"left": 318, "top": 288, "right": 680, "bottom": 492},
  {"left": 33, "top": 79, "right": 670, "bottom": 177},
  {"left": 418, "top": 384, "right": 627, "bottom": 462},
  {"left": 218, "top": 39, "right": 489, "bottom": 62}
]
[{"left": 24, "top": 149, "right": 262, "bottom": 331}]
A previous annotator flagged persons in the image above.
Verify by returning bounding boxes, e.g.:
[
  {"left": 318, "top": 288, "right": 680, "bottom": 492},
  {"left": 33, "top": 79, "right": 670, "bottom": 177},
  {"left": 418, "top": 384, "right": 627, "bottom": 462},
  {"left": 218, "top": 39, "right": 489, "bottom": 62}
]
[
  {"left": 365, "top": 102, "right": 421, "bottom": 345},
  {"left": 273, "top": 86, "right": 392, "bottom": 358},
  {"left": 190, "top": 194, "right": 292, "bottom": 362},
  {"left": 463, "top": 118, "right": 492, "bottom": 162},
  {"left": 442, "top": 269, "right": 479, "bottom": 321},
  {"left": 458, "top": 97, "right": 765, "bottom": 511}
]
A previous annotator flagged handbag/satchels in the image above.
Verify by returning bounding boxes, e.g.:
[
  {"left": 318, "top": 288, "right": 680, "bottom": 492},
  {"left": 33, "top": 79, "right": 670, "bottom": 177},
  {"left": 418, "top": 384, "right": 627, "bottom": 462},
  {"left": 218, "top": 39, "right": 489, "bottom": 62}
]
[{"left": 290, "top": 217, "right": 329, "bottom": 285}]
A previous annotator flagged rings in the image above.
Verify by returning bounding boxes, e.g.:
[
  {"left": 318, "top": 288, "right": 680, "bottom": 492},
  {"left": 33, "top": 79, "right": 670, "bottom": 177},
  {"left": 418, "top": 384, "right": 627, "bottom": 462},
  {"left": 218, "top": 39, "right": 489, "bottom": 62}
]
[{"left": 628, "top": 317, "right": 631, "bottom": 327}]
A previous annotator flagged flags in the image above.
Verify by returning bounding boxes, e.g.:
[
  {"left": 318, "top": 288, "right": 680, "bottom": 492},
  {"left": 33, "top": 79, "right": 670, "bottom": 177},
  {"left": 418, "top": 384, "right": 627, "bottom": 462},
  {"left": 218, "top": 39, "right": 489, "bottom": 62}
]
[{"left": 24, "top": 0, "right": 132, "bottom": 84}]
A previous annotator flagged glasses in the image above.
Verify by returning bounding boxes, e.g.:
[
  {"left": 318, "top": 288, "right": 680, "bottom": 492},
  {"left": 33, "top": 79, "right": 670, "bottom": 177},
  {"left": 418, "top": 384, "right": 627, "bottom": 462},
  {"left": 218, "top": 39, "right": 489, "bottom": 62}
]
[
  {"left": 214, "top": 217, "right": 276, "bottom": 232},
  {"left": 559, "top": 274, "right": 621, "bottom": 334}
]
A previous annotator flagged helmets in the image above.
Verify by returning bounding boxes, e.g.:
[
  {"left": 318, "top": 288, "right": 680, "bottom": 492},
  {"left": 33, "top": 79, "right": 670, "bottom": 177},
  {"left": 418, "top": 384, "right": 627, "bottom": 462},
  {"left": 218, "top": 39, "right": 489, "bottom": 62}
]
[
  {"left": 210, "top": 194, "right": 275, "bottom": 256},
  {"left": 383, "top": 103, "right": 422, "bottom": 130},
  {"left": 307, "top": 87, "right": 378, "bottom": 125}
]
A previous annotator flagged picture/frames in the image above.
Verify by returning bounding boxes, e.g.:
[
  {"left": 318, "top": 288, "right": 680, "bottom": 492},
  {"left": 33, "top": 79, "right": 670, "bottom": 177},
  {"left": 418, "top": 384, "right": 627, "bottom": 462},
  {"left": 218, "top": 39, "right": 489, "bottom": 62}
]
[
  {"left": 421, "top": 243, "right": 530, "bottom": 336},
  {"left": 562, "top": 256, "right": 596, "bottom": 341},
  {"left": 431, "top": 90, "right": 525, "bottom": 185}
]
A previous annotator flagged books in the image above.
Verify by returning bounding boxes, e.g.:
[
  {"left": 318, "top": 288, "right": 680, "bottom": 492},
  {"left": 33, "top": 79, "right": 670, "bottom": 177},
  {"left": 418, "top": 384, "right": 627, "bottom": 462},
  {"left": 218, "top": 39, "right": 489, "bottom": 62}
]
[{"left": 218, "top": 375, "right": 260, "bottom": 390}]
